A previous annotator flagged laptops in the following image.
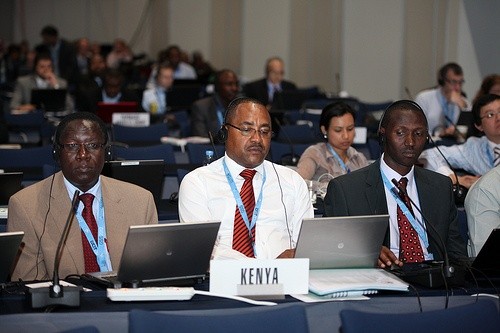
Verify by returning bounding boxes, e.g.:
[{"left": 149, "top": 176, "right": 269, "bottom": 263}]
[
  {"left": 86, "top": 221, "right": 221, "bottom": 289},
  {"left": 101, "top": 160, "right": 165, "bottom": 208},
  {"left": 0, "top": 173, "right": 23, "bottom": 205},
  {"left": 293, "top": 214, "right": 389, "bottom": 269}
]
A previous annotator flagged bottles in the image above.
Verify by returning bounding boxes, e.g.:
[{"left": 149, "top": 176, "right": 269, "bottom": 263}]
[{"left": 203, "top": 150, "right": 215, "bottom": 166}]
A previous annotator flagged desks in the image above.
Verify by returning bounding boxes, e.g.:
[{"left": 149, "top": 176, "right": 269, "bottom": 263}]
[{"left": 0, "top": 295, "right": 500, "bottom": 333}]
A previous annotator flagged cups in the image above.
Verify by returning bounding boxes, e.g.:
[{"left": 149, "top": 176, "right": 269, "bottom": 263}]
[
  {"left": 305, "top": 180, "right": 315, "bottom": 203},
  {"left": 457, "top": 125, "right": 468, "bottom": 142}
]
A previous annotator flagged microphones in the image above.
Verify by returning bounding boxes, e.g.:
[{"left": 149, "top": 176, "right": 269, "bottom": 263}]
[
  {"left": 25, "top": 190, "right": 84, "bottom": 308},
  {"left": 273, "top": 114, "right": 300, "bottom": 165},
  {"left": 391, "top": 179, "right": 460, "bottom": 295},
  {"left": 205, "top": 117, "right": 219, "bottom": 159},
  {"left": 444, "top": 115, "right": 466, "bottom": 139}
]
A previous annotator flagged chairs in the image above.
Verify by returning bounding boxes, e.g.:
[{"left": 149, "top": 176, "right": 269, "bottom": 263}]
[{"left": 0, "top": 87, "right": 500, "bottom": 333}]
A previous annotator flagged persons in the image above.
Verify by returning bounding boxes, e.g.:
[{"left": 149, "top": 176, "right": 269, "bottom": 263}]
[
  {"left": 6, "top": 112, "right": 158, "bottom": 283},
  {"left": 191, "top": 69, "right": 248, "bottom": 146},
  {"left": 297, "top": 102, "right": 368, "bottom": 189},
  {"left": 414, "top": 62, "right": 473, "bottom": 136},
  {"left": 0, "top": 25, "right": 214, "bottom": 114},
  {"left": 178, "top": 98, "right": 316, "bottom": 259},
  {"left": 418, "top": 94, "right": 500, "bottom": 189},
  {"left": 464, "top": 165, "right": 500, "bottom": 258},
  {"left": 242, "top": 58, "right": 299, "bottom": 140},
  {"left": 323, "top": 101, "right": 467, "bottom": 267},
  {"left": 464, "top": 74, "right": 500, "bottom": 143}
]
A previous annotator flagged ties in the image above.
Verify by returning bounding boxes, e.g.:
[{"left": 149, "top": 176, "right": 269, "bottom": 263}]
[
  {"left": 273, "top": 86, "right": 280, "bottom": 103},
  {"left": 232, "top": 169, "right": 258, "bottom": 259},
  {"left": 395, "top": 177, "right": 426, "bottom": 263},
  {"left": 79, "top": 193, "right": 101, "bottom": 274}
]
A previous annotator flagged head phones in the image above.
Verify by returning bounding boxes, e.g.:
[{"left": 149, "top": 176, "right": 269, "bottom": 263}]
[
  {"left": 377, "top": 99, "right": 429, "bottom": 146},
  {"left": 49, "top": 117, "right": 112, "bottom": 161},
  {"left": 218, "top": 96, "right": 246, "bottom": 144}
]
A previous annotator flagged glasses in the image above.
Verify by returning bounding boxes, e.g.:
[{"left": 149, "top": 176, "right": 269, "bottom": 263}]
[
  {"left": 446, "top": 78, "right": 465, "bottom": 86},
  {"left": 480, "top": 112, "right": 499, "bottom": 120},
  {"left": 54, "top": 139, "right": 109, "bottom": 153},
  {"left": 227, "top": 122, "right": 274, "bottom": 139}
]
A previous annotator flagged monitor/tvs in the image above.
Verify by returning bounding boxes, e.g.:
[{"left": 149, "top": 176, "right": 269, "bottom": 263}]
[{"left": 29, "top": 89, "right": 68, "bottom": 119}]
